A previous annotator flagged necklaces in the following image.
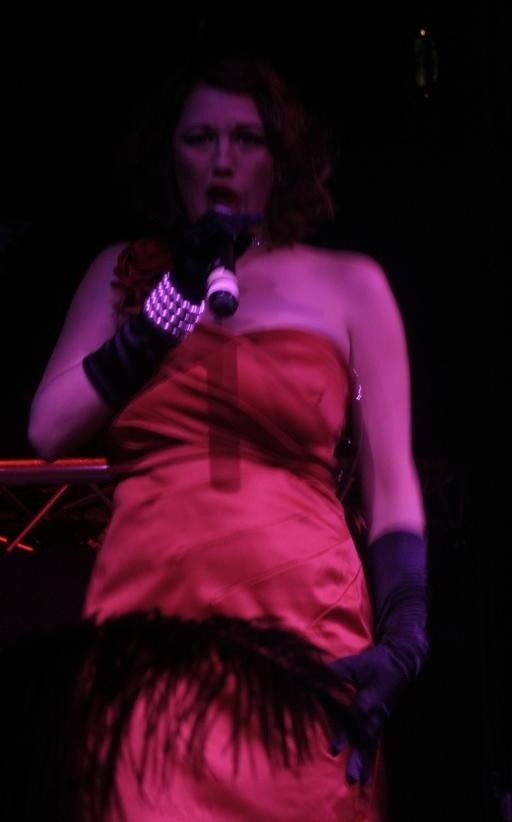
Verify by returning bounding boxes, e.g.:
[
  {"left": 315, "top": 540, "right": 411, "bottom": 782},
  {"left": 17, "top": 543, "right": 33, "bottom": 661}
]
[{"left": 247, "top": 225, "right": 270, "bottom": 249}]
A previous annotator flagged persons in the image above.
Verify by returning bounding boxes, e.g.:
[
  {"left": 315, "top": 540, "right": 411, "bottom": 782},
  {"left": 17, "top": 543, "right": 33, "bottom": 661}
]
[{"left": 26, "top": 54, "right": 430, "bottom": 821}]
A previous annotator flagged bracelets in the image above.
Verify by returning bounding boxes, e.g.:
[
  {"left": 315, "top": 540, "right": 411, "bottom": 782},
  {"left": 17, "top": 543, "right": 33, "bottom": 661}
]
[{"left": 145, "top": 271, "right": 204, "bottom": 339}]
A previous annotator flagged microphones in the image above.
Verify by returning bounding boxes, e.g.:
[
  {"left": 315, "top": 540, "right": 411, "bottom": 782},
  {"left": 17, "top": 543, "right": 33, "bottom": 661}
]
[{"left": 208, "top": 203, "right": 240, "bottom": 314}]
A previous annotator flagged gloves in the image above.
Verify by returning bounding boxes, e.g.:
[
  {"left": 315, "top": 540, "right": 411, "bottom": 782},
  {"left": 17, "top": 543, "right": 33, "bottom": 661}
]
[
  {"left": 329, "top": 531, "right": 430, "bottom": 798},
  {"left": 83, "top": 211, "right": 260, "bottom": 411}
]
